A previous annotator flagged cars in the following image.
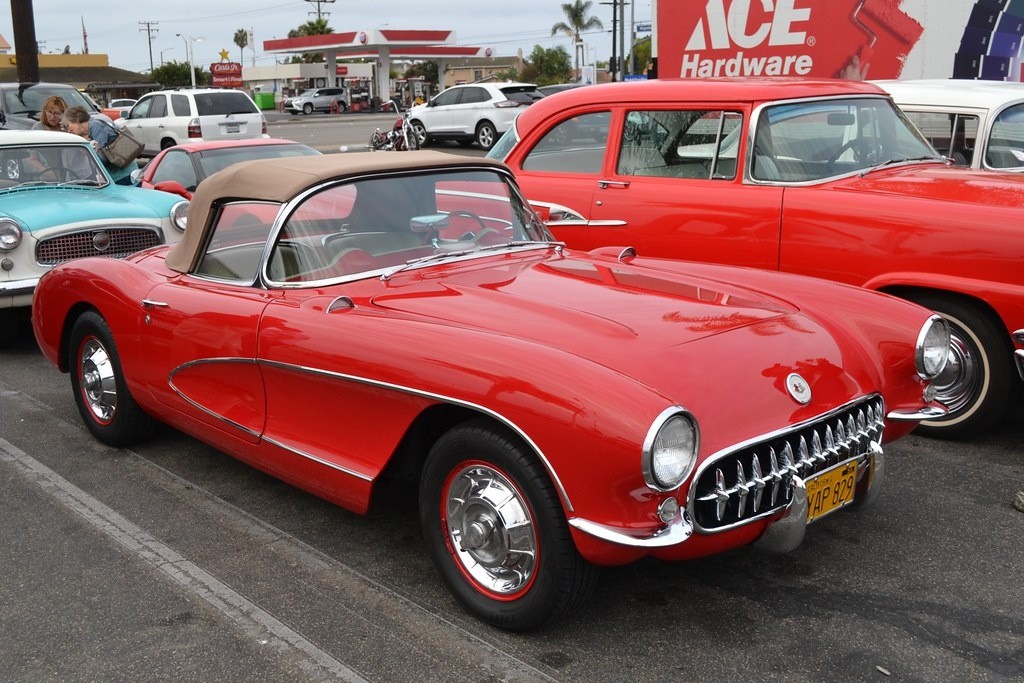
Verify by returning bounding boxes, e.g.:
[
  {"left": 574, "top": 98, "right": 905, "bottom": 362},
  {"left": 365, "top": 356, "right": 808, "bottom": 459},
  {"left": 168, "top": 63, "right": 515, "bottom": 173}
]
[
  {"left": 0, "top": 130, "right": 191, "bottom": 310},
  {"left": 484, "top": 79, "right": 1024, "bottom": 442}
]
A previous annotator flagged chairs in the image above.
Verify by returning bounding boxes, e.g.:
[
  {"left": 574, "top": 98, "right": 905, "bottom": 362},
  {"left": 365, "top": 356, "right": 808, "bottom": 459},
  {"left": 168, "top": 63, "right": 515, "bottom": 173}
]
[
  {"left": 193, "top": 240, "right": 323, "bottom": 285},
  {"left": 632, "top": 164, "right": 717, "bottom": 182},
  {"left": 708, "top": 154, "right": 782, "bottom": 182},
  {"left": 322, "top": 229, "right": 427, "bottom": 265}
]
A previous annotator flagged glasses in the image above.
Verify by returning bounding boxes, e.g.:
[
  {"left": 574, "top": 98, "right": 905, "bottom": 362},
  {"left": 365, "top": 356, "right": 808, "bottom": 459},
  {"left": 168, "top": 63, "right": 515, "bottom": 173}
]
[{"left": 43, "top": 109, "right": 63, "bottom": 117}]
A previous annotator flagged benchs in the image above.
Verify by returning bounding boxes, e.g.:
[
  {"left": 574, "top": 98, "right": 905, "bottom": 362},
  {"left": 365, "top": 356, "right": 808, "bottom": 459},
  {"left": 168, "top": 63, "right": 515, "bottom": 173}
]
[{"left": 521, "top": 144, "right": 669, "bottom": 180}]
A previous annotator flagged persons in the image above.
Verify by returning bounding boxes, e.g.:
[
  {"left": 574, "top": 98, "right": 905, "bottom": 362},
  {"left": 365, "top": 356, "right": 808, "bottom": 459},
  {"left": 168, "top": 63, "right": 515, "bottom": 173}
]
[{"left": 28, "top": 96, "right": 146, "bottom": 185}]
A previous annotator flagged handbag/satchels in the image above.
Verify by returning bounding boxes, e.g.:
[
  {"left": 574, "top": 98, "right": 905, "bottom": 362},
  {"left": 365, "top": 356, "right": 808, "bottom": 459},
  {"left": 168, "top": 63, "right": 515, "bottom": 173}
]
[{"left": 89, "top": 119, "right": 146, "bottom": 171}]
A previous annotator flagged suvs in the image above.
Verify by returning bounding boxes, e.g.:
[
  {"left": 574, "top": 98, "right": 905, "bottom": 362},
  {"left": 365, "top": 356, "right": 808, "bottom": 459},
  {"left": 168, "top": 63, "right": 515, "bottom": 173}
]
[
  {"left": 284, "top": 88, "right": 348, "bottom": 114},
  {"left": 406, "top": 82, "right": 548, "bottom": 151},
  {"left": 113, "top": 89, "right": 269, "bottom": 158}
]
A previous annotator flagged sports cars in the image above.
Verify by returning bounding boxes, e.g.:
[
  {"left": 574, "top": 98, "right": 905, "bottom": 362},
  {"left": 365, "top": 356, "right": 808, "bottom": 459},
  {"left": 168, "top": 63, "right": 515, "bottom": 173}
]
[
  {"left": 131, "top": 138, "right": 359, "bottom": 245},
  {"left": 32, "top": 152, "right": 951, "bottom": 634}
]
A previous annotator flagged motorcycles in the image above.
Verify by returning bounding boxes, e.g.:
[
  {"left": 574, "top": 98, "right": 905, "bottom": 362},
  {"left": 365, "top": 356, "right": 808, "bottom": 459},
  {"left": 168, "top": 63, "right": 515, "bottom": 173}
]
[{"left": 364, "top": 99, "right": 420, "bottom": 152}]
